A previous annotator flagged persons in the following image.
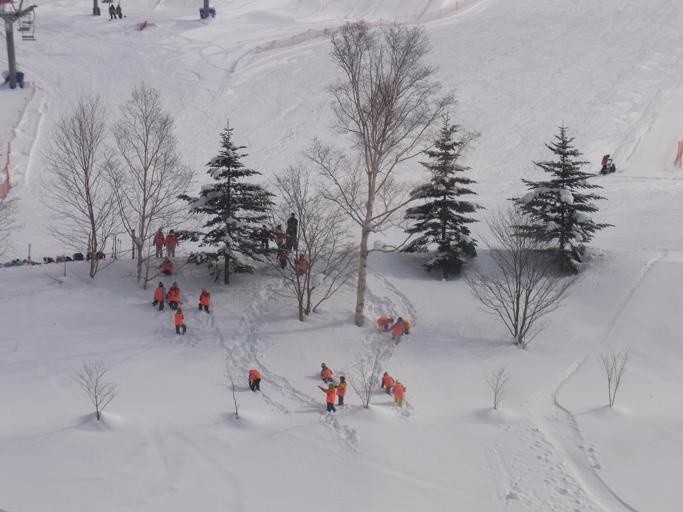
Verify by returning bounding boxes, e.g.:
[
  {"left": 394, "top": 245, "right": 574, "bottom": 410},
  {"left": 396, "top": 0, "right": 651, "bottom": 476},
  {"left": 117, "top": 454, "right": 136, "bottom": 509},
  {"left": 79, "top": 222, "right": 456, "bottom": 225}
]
[
  {"left": 276, "top": 247, "right": 288, "bottom": 268},
  {"left": 377, "top": 315, "right": 393, "bottom": 332},
  {"left": 248, "top": 368, "right": 261, "bottom": 392},
  {"left": 164, "top": 229, "right": 178, "bottom": 258},
  {"left": 397, "top": 317, "right": 410, "bottom": 335},
  {"left": 198, "top": 288, "right": 210, "bottom": 314},
  {"left": 109, "top": 3, "right": 116, "bottom": 20},
  {"left": 391, "top": 381, "right": 406, "bottom": 407},
  {"left": 116, "top": 4, "right": 122, "bottom": 18},
  {"left": 260, "top": 224, "right": 271, "bottom": 249},
  {"left": 599, "top": 153, "right": 610, "bottom": 174},
  {"left": 317, "top": 383, "right": 336, "bottom": 413},
  {"left": 0, "top": 70, "right": 24, "bottom": 89},
  {"left": 166, "top": 280, "right": 184, "bottom": 310},
  {"left": 285, "top": 213, "right": 297, "bottom": 251},
  {"left": 320, "top": 362, "right": 334, "bottom": 383},
  {"left": 293, "top": 254, "right": 310, "bottom": 276},
  {"left": 273, "top": 224, "right": 284, "bottom": 248},
  {"left": 334, "top": 376, "right": 346, "bottom": 405},
  {"left": 391, "top": 324, "right": 401, "bottom": 344},
  {"left": 160, "top": 256, "right": 173, "bottom": 275},
  {"left": 174, "top": 308, "right": 185, "bottom": 335},
  {"left": 152, "top": 227, "right": 164, "bottom": 256},
  {"left": 380, "top": 371, "right": 394, "bottom": 396},
  {"left": 152, "top": 282, "right": 166, "bottom": 311}
]
